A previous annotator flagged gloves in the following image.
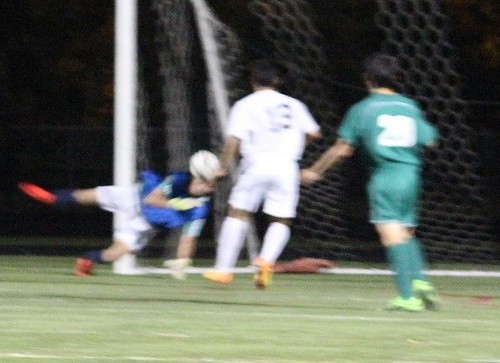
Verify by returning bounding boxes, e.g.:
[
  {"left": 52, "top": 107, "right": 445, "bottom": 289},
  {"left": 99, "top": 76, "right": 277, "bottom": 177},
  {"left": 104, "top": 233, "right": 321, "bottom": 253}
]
[
  {"left": 176, "top": 259, "right": 200, "bottom": 274},
  {"left": 171, "top": 197, "right": 202, "bottom": 210}
]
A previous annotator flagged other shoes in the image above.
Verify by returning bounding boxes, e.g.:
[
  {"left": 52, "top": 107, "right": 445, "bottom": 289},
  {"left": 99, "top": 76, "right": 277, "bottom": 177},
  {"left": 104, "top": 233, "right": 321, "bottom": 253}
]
[
  {"left": 413, "top": 280, "right": 442, "bottom": 311},
  {"left": 78, "top": 252, "right": 95, "bottom": 276},
  {"left": 19, "top": 183, "right": 57, "bottom": 208},
  {"left": 253, "top": 265, "right": 275, "bottom": 288},
  {"left": 202, "top": 267, "right": 234, "bottom": 283},
  {"left": 382, "top": 296, "right": 423, "bottom": 313}
]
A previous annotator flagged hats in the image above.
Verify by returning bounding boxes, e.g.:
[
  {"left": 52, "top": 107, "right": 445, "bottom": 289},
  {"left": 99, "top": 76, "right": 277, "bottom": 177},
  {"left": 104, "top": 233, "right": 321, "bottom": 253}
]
[{"left": 189, "top": 150, "right": 227, "bottom": 180}]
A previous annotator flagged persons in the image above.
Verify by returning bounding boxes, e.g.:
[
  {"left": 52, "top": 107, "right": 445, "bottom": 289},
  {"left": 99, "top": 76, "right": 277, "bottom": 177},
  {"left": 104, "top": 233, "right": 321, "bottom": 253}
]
[
  {"left": 204, "top": 66, "right": 325, "bottom": 289},
  {"left": 301, "top": 54, "right": 442, "bottom": 312},
  {"left": 18, "top": 149, "right": 223, "bottom": 275}
]
